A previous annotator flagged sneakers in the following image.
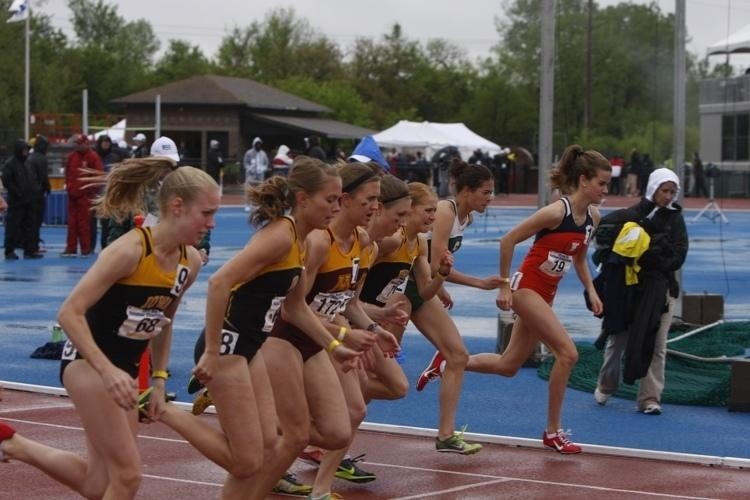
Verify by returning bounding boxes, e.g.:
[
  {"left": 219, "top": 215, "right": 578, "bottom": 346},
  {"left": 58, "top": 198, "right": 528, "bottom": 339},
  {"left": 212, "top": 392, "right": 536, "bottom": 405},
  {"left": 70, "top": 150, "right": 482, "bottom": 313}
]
[
  {"left": 542, "top": 428, "right": 582, "bottom": 454},
  {"left": 268, "top": 471, "right": 314, "bottom": 498},
  {"left": 138, "top": 385, "right": 155, "bottom": 424},
  {"left": 59, "top": 252, "right": 94, "bottom": 259},
  {"left": 416, "top": 349, "right": 447, "bottom": 392},
  {"left": 305, "top": 491, "right": 344, "bottom": 500},
  {"left": 334, "top": 455, "right": 377, "bottom": 484},
  {"left": 594, "top": 387, "right": 612, "bottom": 406},
  {"left": 636, "top": 400, "right": 663, "bottom": 415},
  {"left": 0, "top": 421, "right": 17, "bottom": 464},
  {"left": 4, "top": 241, "right": 47, "bottom": 259},
  {"left": 187, "top": 374, "right": 217, "bottom": 416},
  {"left": 435, "top": 433, "right": 483, "bottom": 456},
  {"left": 296, "top": 444, "right": 328, "bottom": 469}
]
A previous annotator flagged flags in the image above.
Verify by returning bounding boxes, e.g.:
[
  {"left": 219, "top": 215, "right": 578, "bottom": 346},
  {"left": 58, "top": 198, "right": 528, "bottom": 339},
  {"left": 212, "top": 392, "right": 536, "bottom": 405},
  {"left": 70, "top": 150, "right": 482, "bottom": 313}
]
[{"left": 5, "top": 0, "right": 30, "bottom": 23}]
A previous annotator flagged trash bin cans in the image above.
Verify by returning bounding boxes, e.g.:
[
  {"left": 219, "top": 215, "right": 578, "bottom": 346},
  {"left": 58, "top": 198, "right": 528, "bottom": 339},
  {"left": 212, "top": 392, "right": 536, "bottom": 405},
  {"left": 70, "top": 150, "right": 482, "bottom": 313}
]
[{"left": 621, "top": 164, "right": 639, "bottom": 198}]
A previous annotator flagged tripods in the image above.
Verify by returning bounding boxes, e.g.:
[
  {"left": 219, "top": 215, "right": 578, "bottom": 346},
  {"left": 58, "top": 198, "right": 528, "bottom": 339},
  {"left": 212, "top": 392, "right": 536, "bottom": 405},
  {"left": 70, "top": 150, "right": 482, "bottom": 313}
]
[{"left": 692, "top": 197, "right": 729, "bottom": 227}]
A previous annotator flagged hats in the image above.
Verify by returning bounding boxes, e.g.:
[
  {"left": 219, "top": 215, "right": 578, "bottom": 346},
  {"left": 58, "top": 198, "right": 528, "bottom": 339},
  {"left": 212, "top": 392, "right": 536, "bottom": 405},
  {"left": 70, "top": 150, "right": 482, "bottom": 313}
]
[
  {"left": 347, "top": 155, "right": 372, "bottom": 163},
  {"left": 73, "top": 134, "right": 89, "bottom": 145},
  {"left": 149, "top": 136, "right": 181, "bottom": 163},
  {"left": 133, "top": 133, "right": 147, "bottom": 144}
]
